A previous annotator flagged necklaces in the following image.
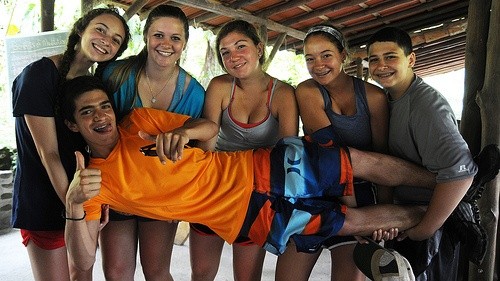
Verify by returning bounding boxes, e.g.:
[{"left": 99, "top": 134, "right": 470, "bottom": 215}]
[{"left": 145, "top": 61, "right": 176, "bottom": 103}]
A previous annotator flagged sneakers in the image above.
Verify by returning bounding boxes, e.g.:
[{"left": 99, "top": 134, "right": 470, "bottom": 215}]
[
  {"left": 479, "top": 233, "right": 481, "bottom": 238},
  {"left": 444, "top": 201, "right": 489, "bottom": 266},
  {"left": 462, "top": 144, "right": 500, "bottom": 204}
]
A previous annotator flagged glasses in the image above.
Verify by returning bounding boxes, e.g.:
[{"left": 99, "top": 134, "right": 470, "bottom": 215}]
[{"left": 138, "top": 142, "right": 160, "bottom": 157}]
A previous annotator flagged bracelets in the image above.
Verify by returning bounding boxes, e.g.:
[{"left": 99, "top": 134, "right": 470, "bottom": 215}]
[{"left": 61, "top": 207, "right": 86, "bottom": 221}]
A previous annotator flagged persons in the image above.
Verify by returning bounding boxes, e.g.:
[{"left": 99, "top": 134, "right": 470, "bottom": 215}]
[
  {"left": 58, "top": 74, "right": 500, "bottom": 272},
  {"left": 10, "top": 8, "right": 132, "bottom": 281},
  {"left": 275, "top": 23, "right": 389, "bottom": 281},
  {"left": 94, "top": 4, "right": 206, "bottom": 281},
  {"left": 189, "top": 19, "right": 300, "bottom": 281},
  {"left": 352, "top": 27, "right": 479, "bottom": 281}
]
